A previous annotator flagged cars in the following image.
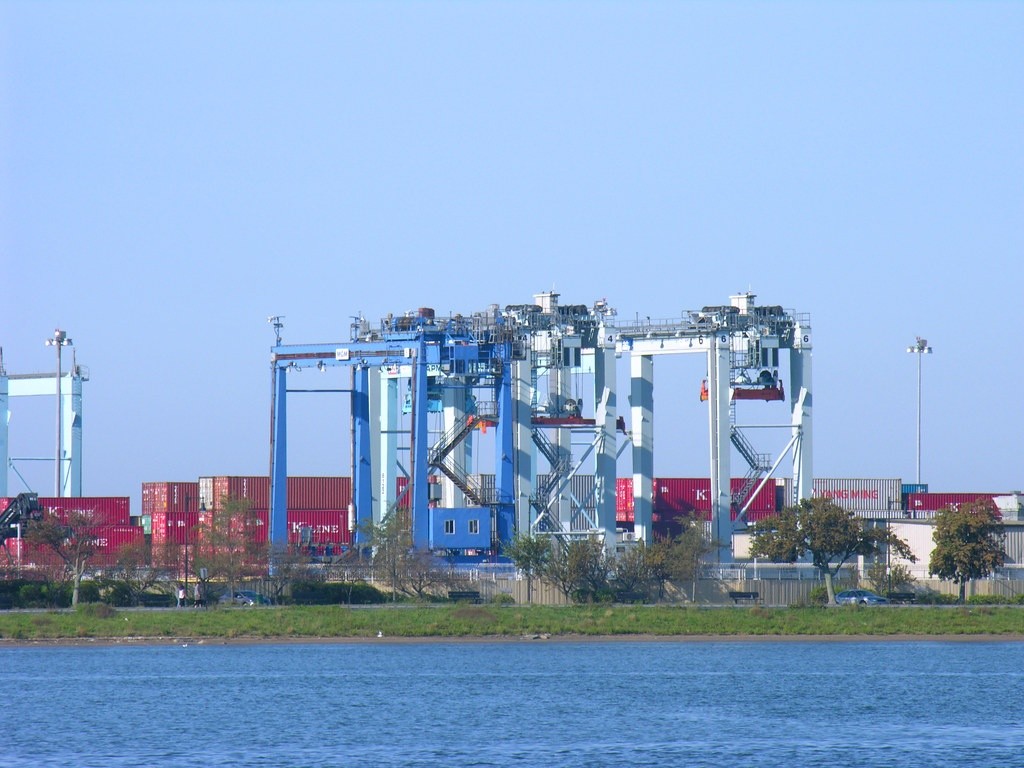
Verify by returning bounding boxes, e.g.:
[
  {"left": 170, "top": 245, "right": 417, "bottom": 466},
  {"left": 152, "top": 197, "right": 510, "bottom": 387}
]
[
  {"left": 218, "top": 590, "right": 272, "bottom": 605},
  {"left": 834, "top": 589, "right": 889, "bottom": 606}
]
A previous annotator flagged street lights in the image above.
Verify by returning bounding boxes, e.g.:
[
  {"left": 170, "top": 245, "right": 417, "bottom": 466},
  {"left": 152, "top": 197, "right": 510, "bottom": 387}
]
[
  {"left": 527, "top": 495, "right": 547, "bottom": 605},
  {"left": 887, "top": 496, "right": 908, "bottom": 604},
  {"left": 906, "top": 337, "right": 933, "bottom": 485},
  {"left": 44, "top": 329, "right": 74, "bottom": 498},
  {"left": 10, "top": 523, "right": 21, "bottom": 581},
  {"left": 184, "top": 492, "right": 207, "bottom": 608}
]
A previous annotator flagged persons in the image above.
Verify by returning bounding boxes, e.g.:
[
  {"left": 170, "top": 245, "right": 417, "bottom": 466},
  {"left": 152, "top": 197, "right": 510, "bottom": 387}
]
[
  {"left": 177, "top": 583, "right": 189, "bottom": 608},
  {"left": 194, "top": 581, "right": 206, "bottom": 608}
]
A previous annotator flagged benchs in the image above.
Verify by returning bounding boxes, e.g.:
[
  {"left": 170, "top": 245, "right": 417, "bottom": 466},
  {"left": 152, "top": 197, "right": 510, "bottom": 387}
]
[
  {"left": 448, "top": 591, "right": 483, "bottom": 605},
  {"left": 617, "top": 591, "right": 649, "bottom": 605},
  {"left": 887, "top": 592, "right": 919, "bottom": 605},
  {"left": 728, "top": 592, "right": 763, "bottom": 604}
]
